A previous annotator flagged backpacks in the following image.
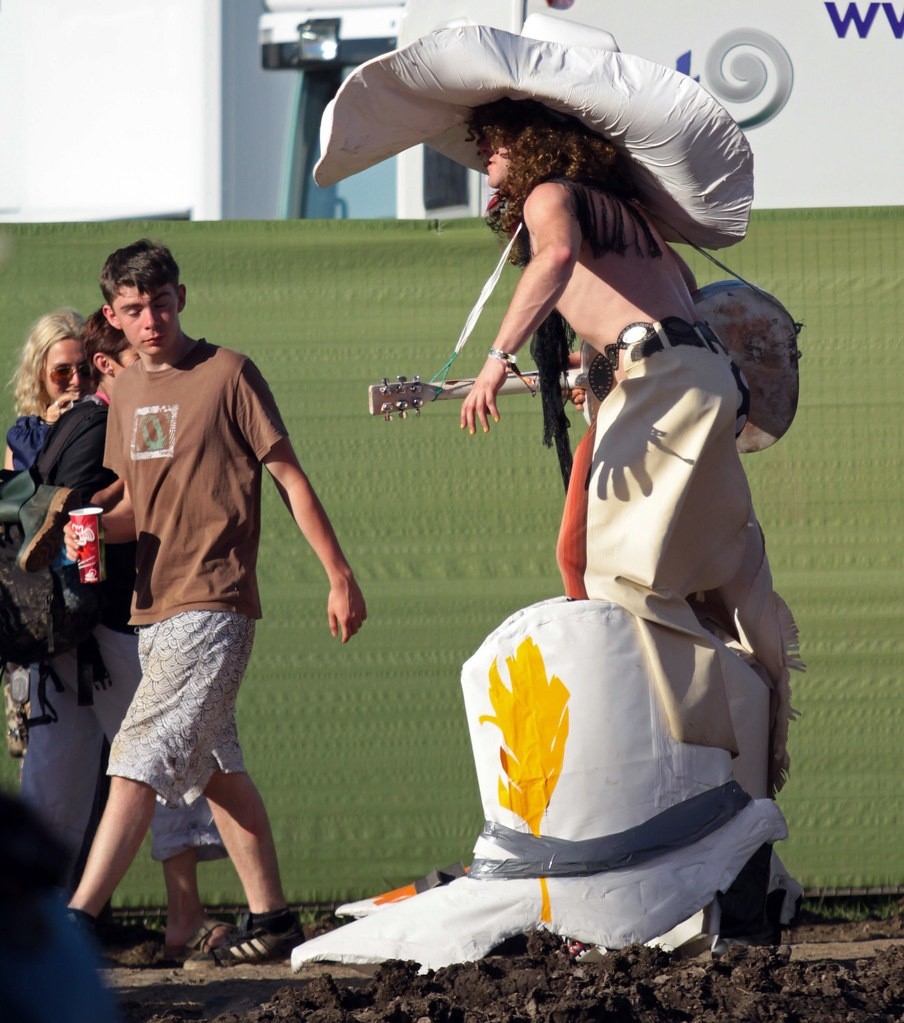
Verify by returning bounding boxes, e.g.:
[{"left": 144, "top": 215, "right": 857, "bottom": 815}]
[{"left": 0, "top": 403, "right": 110, "bottom": 665}]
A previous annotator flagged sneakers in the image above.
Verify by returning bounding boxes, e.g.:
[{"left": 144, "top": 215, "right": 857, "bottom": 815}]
[{"left": 183, "top": 901, "right": 306, "bottom": 973}]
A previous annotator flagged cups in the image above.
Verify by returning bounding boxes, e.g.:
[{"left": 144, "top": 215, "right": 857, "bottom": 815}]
[{"left": 68, "top": 507, "right": 106, "bottom": 583}]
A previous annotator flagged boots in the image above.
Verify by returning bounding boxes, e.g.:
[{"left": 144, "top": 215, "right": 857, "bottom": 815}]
[{"left": 1, "top": 463, "right": 83, "bottom": 571}]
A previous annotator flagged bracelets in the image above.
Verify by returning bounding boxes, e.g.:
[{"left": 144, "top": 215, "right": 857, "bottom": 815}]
[{"left": 489, "top": 347, "right": 517, "bottom": 373}]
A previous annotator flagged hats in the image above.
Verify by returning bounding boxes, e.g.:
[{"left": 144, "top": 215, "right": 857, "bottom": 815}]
[{"left": 314, "top": 18, "right": 753, "bottom": 249}]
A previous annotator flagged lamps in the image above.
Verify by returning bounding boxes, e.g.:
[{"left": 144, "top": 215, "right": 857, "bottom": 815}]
[{"left": 295, "top": 15, "right": 341, "bottom": 61}]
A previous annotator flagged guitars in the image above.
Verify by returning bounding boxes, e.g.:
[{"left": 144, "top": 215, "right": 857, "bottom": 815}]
[{"left": 367, "top": 280, "right": 800, "bottom": 455}]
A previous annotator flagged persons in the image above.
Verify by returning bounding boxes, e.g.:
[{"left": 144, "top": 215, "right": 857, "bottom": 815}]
[
  {"left": 20, "top": 306, "right": 232, "bottom": 959},
  {"left": 66, "top": 238, "right": 369, "bottom": 969},
  {"left": 0, "top": 311, "right": 115, "bottom": 924},
  {"left": 460, "top": 92, "right": 798, "bottom": 797}
]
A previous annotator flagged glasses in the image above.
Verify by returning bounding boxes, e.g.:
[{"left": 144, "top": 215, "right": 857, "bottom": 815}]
[{"left": 45, "top": 365, "right": 99, "bottom": 383}]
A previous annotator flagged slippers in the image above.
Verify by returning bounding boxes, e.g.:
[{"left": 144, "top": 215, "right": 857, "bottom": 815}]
[{"left": 163, "top": 909, "right": 230, "bottom": 965}]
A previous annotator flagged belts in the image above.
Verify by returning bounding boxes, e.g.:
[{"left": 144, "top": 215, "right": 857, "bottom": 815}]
[{"left": 633, "top": 328, "right": 716, "bottom": 361}]
